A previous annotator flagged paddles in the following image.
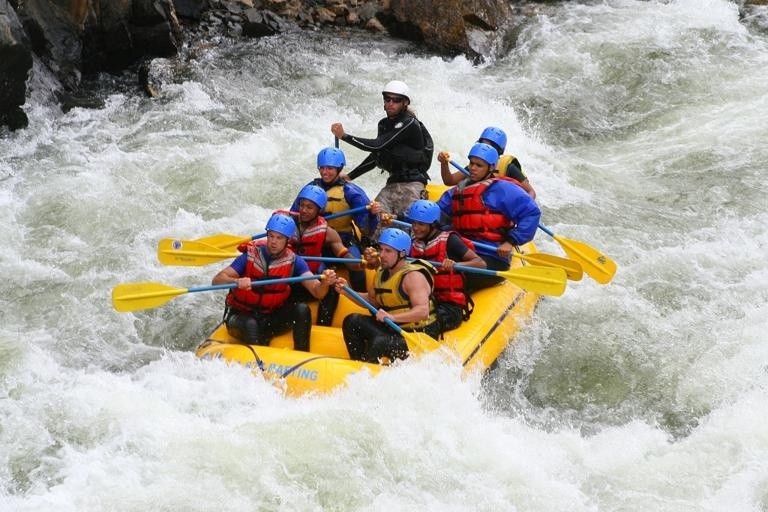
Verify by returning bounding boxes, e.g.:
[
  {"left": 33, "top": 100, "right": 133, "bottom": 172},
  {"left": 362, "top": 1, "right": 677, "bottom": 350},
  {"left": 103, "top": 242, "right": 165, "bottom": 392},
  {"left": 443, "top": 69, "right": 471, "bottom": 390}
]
[
  {"left": 197, "top": 199, "right": 377, "bottom": 252},
  {"left": 370, "top": 250, "right": 566, "bottom": 297},
  {"left": 112, "top": 271, "right": 330, "bottom": 313},
  {"left": 443, "top": 153, "right": 618, "bottom": 284},
  {"left": 157, "top": 238, "right": 242, "bottom": 265},
  {"left": 338, "top": 282, "right": 444, "bottom": 358},
  {"left": 382, "top": 212, "right": 582, "bottom": 282}
]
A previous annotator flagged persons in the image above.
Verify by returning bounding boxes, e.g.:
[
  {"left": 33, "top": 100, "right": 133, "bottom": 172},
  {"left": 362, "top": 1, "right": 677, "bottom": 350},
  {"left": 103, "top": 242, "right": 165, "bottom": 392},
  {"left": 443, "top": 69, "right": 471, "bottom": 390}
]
[
  {"left": 335, "top": 228, "right": 439, "bottom": 366},
  {"left": 407, "top": 199, "right": 487, "bottom": 332},
  {"left": 435, "top": 143, "right": 541, "bottom": 293},
  {"left": 273, "top": 184, "right": 366, "bottom": 329},
  {"left": 291, "top": 148, "right": 382, "bottom": 292},
  {"left": 331, "top": 80, "right": 434, "bottom": 218},
  {"left": 438, "top": 127, "right": 536, "bottom": 200},
  {"left": 212, "top": 214, "right": 336, "bottom": 355}
]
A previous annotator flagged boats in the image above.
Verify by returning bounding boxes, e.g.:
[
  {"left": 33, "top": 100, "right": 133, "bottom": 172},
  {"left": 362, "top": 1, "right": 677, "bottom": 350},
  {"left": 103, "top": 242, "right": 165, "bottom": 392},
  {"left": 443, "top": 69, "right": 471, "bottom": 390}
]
[{"left": 191, "top": 182, "right": 544, "bottom": 402}]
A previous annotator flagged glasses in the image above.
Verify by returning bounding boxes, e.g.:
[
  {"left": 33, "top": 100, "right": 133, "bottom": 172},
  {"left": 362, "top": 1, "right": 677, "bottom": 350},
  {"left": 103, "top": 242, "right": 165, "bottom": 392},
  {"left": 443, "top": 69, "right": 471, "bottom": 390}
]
[{"left": 384, "top": 94, "right": 403, "bottom": 103}]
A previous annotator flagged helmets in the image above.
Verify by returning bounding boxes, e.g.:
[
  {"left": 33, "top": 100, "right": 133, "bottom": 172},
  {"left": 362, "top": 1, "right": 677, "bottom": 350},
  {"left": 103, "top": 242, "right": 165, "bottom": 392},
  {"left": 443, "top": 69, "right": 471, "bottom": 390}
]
[
  {"left": 468, "top": 143, "right": 498, "bottom": 166},
  {"left": 265, "top": 148, "right": 346, "bottom": 258},
  {"left": 407, "top": 200, "right": 441, "bottom": 225},
  {"left": 480, "top": 127, "right": 506, "bottom": 153},
  {"left": 382, "top": 81, "right": 410, "bottom": 104},
  {"left": 378, "top": 228, "right": 412, "bottom": 255}
]
